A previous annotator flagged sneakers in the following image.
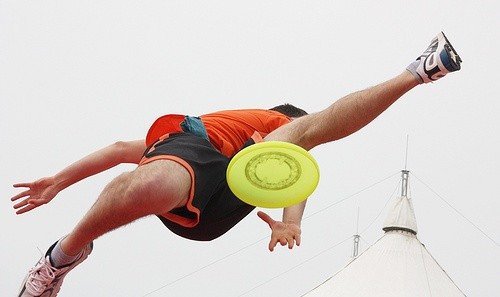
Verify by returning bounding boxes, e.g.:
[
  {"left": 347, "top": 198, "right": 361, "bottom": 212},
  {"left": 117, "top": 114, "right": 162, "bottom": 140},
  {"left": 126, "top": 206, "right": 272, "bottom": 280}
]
[
  {"left": 415, "top": 30, "right": 463, "bottom": 81},
  {"left": 18, "top": 238, "right": 94, "bottom": 296}
]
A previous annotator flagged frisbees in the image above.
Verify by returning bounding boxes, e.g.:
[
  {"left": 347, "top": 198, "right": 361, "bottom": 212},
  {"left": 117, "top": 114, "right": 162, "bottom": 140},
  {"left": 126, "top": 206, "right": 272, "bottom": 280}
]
[{"left": 224, "top": 140, "right": 321, "bottom": 211}]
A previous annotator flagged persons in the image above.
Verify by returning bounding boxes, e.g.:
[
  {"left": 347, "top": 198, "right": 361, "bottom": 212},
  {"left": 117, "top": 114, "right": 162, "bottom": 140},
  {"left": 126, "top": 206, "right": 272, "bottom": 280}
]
[{"left": 10, "top": 30, "right": 463, "bottom": 297}]
